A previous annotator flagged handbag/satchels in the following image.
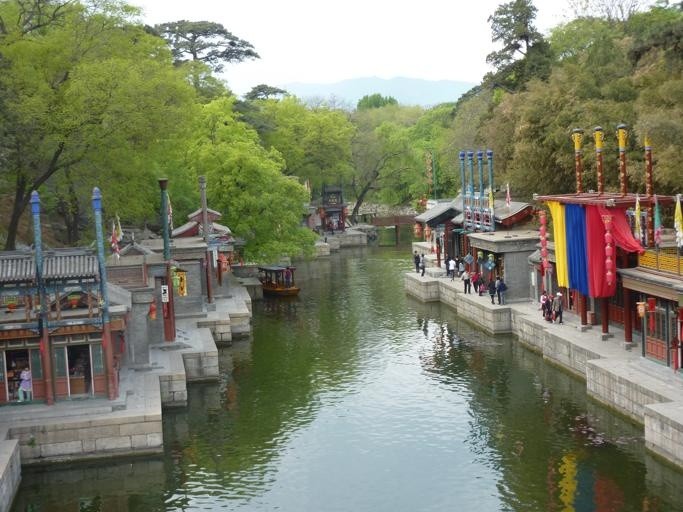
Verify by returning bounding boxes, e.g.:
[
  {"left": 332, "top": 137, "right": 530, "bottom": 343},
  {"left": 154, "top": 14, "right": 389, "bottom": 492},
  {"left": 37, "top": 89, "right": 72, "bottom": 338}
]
[{"left": 479, "top": 285, "right": 487, "bottom": 292}]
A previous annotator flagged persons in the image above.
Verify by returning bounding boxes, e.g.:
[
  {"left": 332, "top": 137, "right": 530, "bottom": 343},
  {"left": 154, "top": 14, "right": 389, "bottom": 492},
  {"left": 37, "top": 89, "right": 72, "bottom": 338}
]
[
  {"left": 540, "top": 291, "right": 563, "bottom": 325},
  {"left": 17, "top": 365, "right": 32, "bottom": 402},
  {"left": 444, "top": 256, "right": 508, "bottom": 305},
  {"left": 414, "top": 251, "right": 425, "bottom": 276}
]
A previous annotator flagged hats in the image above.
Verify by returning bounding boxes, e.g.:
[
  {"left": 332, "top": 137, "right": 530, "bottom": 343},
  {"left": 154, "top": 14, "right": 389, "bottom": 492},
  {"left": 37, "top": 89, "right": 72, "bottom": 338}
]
[{"left": 556, "top": 292, "right": 563, "bottom": 297}]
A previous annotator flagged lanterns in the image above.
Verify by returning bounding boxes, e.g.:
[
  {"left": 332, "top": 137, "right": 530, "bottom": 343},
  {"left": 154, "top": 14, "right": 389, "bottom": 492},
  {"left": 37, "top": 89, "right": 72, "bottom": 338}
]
[
  {"left": 604, "top": 219, "right": 613, "bottom": 285},
  {"left": 540, "top": 210, "right": 549, "bottom": 270}
]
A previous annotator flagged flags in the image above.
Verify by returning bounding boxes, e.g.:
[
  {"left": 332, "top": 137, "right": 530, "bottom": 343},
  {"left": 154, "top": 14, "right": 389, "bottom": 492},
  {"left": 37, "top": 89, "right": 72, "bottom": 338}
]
[
  {"left": 674, "top": 196, "right": 683, "bottom": 247},
  {"left": 634, "top": 194, "right": 642, "bottom": 242},
  {"left": 654, "top": 194, "right": 664, "bottom": 247}
]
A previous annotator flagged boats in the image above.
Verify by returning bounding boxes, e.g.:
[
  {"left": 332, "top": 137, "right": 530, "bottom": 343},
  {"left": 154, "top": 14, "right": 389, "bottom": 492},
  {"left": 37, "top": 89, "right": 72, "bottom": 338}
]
[{"left": 258, "top": 264, "right": 300, "bottom": 295}]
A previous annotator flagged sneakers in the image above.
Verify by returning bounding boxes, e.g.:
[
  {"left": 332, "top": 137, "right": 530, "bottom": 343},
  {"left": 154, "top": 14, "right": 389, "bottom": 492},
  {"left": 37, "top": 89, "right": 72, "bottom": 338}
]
[{"left": 542, "top": 316, "right": 563, "bottom": 325}]
